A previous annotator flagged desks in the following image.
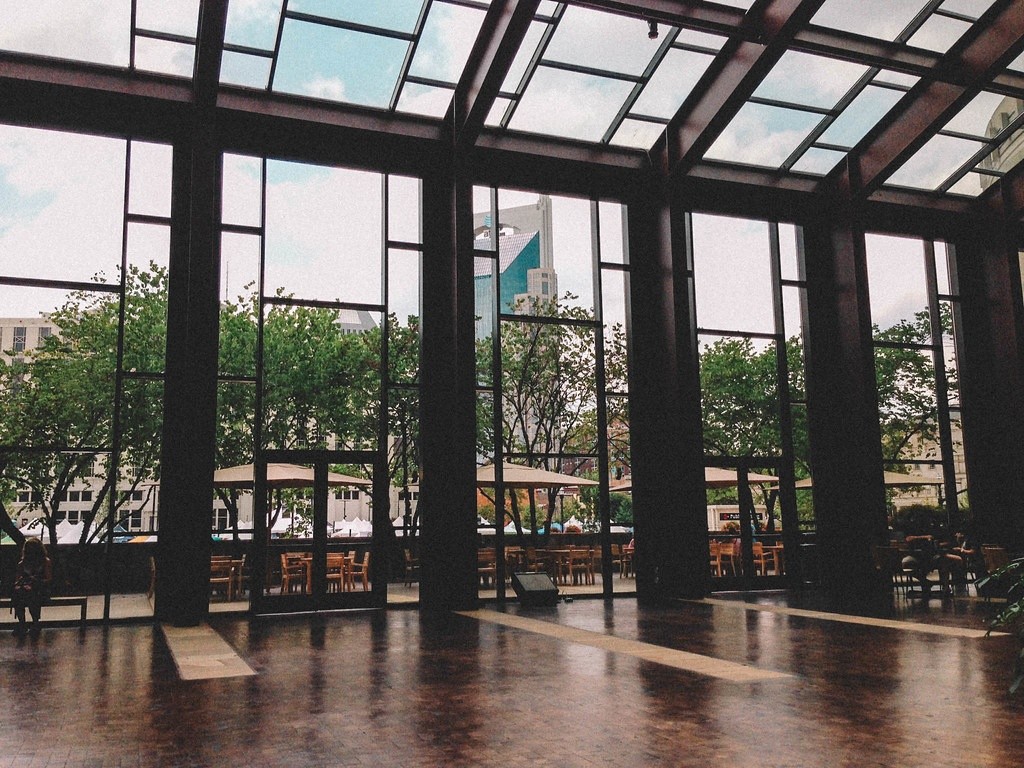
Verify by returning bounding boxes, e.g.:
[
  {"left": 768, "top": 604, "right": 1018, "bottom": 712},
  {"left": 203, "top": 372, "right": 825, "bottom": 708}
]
[
  {"left": 535, "top": 549, "right": 596, "bottom": 586},
  {"left": 210, "top": 560, "right": 243, "bottom": 600},
  {"left": 299, "top": 557, "right": 351, "bottom": 593},
  {"left": 507, "top": 549, "right": 529, "bottom": 572},
  {"left": 763, "top": 545, "right": 784, "bottom": 575}
]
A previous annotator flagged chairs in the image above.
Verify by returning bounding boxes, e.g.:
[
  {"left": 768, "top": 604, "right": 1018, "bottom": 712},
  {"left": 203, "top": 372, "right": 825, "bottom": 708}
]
[{"left": 147, "top": 538, "right": 1007, "bottom": 600}]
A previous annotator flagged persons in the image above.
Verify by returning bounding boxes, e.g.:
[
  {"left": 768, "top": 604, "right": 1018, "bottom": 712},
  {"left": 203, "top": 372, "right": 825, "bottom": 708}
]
[
  {"left": 627, "top": 538, "right": 635, "bottom": 554},
  {"left": 0, "top": 501, "right": 26, "bottom": 545},
  {"left": 12, "top": 537, "right": 54, "bottom": 637},
  {"left": 728, "top": 528, "right": 741, "bottom": 553},
  {"left": 901, "top": 514, "right": 990, "bottom": 597}
]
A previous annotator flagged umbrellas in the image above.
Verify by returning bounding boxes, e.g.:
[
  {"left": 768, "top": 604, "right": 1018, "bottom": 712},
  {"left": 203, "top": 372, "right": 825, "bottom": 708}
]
[
  {"left": 762, "top": 469, "right": 945, "bottom": 489},
  {"left": 213, "top": 463, "right": 372, "bottom": 526},
  {"left": 608, "top": 467, "right": 778, "bottom": 492},
  {"left": 405, "top": 461, "right": 599, "bottom": 490}
]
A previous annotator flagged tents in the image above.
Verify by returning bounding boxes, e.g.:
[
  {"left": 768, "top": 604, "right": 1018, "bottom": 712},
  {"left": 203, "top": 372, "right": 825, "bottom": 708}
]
[{"left": 18, "top": 517, "right": 105, "bottom": 543}]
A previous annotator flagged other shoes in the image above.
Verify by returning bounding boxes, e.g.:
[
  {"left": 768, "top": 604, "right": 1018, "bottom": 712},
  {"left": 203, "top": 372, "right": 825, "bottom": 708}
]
[
  {"left": 15, "top": 623, "right": 25, "bottom": 632},
  {"left": 32, "top": 623, "right": 41, "bottom": 632}
]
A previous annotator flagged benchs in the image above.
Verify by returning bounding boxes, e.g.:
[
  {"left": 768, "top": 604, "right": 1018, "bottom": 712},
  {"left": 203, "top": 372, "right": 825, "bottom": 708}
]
[{"left": 0, "top": 596, "right": 87, "bottom": 631}]
